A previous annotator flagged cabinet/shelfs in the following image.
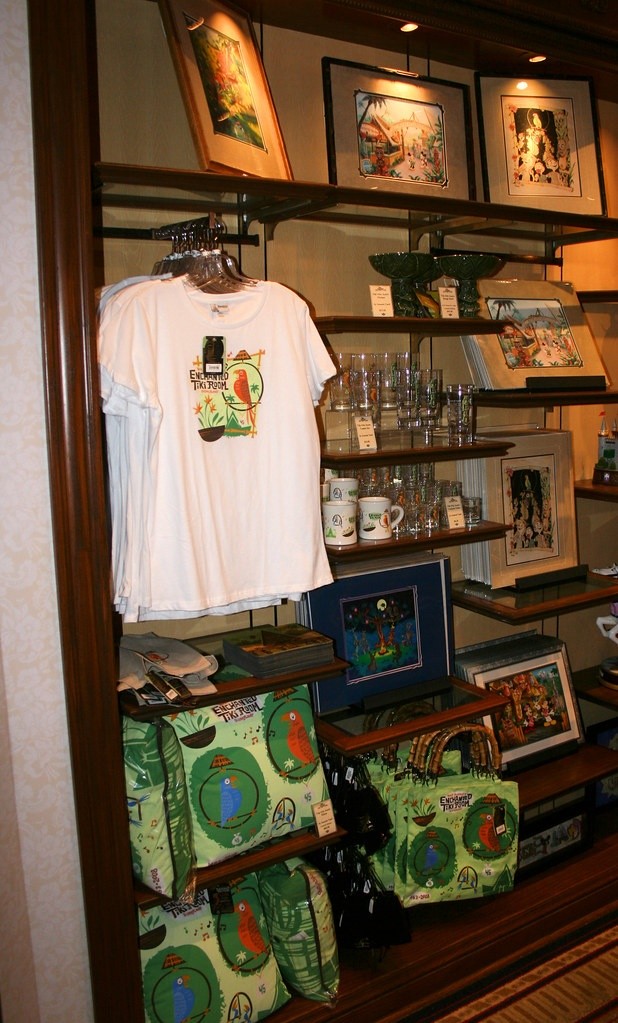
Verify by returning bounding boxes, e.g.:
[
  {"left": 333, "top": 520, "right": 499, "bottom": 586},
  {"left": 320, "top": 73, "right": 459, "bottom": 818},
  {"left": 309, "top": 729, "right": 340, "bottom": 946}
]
[{"left": 26, "top": 0, "right": 618, "bottom": 1023}]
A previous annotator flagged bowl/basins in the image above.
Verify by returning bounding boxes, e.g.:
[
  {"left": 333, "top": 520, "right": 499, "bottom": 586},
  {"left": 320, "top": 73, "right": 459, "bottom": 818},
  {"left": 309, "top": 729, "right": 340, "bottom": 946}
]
[{"left": 369, "top": 252, "right": 502, "bottom": 320}]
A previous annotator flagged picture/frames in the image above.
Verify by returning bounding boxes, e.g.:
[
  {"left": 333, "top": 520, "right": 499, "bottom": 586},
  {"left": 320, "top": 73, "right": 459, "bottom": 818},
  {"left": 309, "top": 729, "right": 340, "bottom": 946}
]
[
  {"left": 158, "top": 0, "right": 294, "bottom": 181},
  {"left": 321, "top": 56, "right": 477, "bottom": 201},
  {"left": 453, "top": 633, "right": 586, "bottom": 774},
  {"left": 474, "top": 72, "right": 608, "bottom": 217}
]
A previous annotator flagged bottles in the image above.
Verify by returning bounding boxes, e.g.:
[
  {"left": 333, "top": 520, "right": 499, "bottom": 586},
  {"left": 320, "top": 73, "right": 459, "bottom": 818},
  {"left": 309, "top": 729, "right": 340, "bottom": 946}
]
[{"left": 598, "top": 417, "right": 618, "bottom": 470}]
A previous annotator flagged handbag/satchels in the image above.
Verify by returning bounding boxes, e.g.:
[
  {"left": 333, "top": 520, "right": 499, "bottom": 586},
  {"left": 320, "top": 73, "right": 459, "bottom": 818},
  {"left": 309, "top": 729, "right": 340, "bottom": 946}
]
[{"left": 318, "top": 723, "right": 524, "bottom": 946}]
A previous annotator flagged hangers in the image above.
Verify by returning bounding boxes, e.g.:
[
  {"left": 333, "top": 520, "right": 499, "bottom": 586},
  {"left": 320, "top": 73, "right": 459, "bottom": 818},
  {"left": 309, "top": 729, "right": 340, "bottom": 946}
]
[{"left": 150, "top": 215, "right": 261, "bottom": 297}]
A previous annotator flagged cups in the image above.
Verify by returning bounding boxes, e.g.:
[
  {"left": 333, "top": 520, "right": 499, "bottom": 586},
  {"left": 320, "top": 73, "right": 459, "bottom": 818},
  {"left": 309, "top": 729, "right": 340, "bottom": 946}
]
[
  {"left": 320, "top": 467, "right": 482, "bottom": 545},
  {"left": 328, "top": 351, "right": 474, "bottom": 445}
]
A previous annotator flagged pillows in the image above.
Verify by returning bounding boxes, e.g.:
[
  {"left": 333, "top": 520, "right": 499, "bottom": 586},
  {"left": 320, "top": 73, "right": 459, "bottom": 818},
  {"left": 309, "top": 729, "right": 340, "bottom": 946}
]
[
  {"left": 259, "top": 857, "right": 341, "bottom": 1003},
  {"left": 121, "top": 715, "right": 192, "bottom": 900},
  {"left": 138, "top": 872, "right": 292, "bottom": 1023},
  {"left": 158, "top": 684, "right": 331, "bottom": 868}
]
